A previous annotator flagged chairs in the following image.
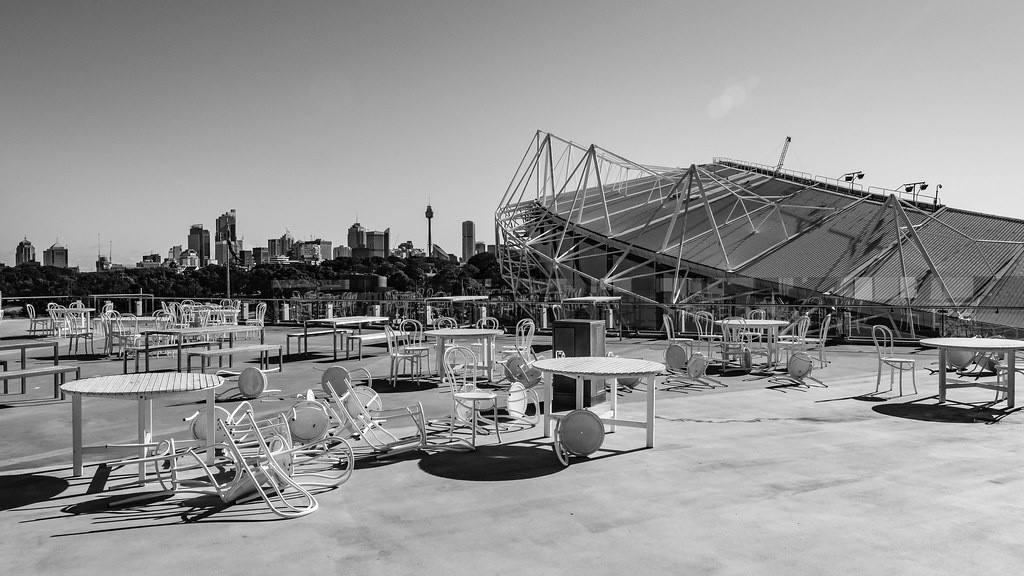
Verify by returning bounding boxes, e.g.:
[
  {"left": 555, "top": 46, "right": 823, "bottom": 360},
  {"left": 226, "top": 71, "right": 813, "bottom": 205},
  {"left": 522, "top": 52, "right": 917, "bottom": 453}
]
[
  {"left": 871, "top": 325, "right": 918, "bottom": 398},
  {"left": 660, "top": 344, "right": 728, "bottom": 390},
  {"left": 27, "top": 302, "right": 114, "bottom": 356},
  {"left": 369, "top": 316, "right": 549, "bottom": 451},
  {"left": 105, "top": 366, "right": 397, "bottom": 554},
  {"left": 547, "top": 410, "right": 606, "bottom": 466},
  {"left": 992, "top": 363, "right": 1024, "bottom": 403},
  {"left": 663, "top": 314, "right": 693, "bottom": 363},
  {"left": 767, "top": 352, "right": 829, "bottom": 390},
  {"left": 101, "top": 300, "right": 267, "bottom": 360},
  {"left": 693, "top": 310, "right": 831, "bottom": 372},
  {"left": 606, "top": 352, "right": 658, "bottom": 396}
]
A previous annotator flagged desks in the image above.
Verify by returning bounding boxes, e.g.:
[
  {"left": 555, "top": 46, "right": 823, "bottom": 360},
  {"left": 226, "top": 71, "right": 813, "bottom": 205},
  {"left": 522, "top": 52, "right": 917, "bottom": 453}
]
[
  {"left": 93, "top": 315, "right": 170, "bottom": 352},
  {"left": 130, "top": 340, "right": 224, "bottom": 372},
  {"left": 60, "top": 372, "right": 225, "bottom": 486},
  {"left": 47, "top": 308, "right": 95, "bottom": 336},
  {"left": 532, "top": 356, "right": 666, "bottom": 447},
  {"left": 919, "top": 337, "right": 1024, "bottom": 407},
  {"left": 145, "top": 325, "right": 265, "bottom": 371},
  {"left": 302, "top": 314, "right": 389, "bottom": 361},
  {"left": 195, "top": 309, "right": 241, "bottom": 327},
  {"left": 424, "top": 328, "right": 504, "bottom": 382},
  {"left": 716, "top": 319, "right": 790, "bottom": 366}
]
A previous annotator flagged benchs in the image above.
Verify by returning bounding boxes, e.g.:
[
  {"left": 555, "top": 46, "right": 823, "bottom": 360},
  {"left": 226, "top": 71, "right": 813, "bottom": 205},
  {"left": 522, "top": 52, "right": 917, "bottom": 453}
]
[
  {"left": 0, "top": 364, "right": 80, "bottom": 400},
  {"left": 285, "top": 329, "right": 353, "bottom": 356},
  {"left": 187, "top": 345, "right": 284, "bottom": 373},
  {"left": 344, "top": 330, "right": 412, "bottom": 362}
]
